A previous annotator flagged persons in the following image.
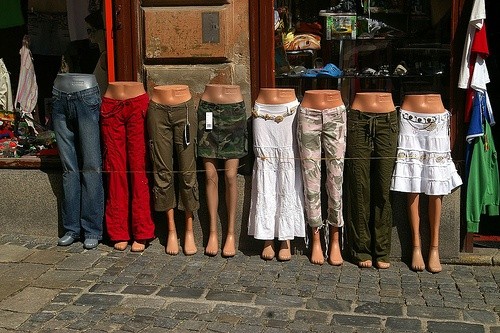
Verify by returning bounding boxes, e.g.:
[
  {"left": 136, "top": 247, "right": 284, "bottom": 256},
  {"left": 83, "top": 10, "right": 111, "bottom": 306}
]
[
  {"left": 52, "top": 73, "right": 105, "bottom": 248},
  {"left": 84, "top": 9, "right": 107, "bottom": 96},
  {"left": 147, "top": 85, "right": 200, "bottom": 254},
  {"left": 389, "top": 94, "right": 463, "bottom": 273},
  {"left": 296, "top": 90, "right": 347, "bottom": 264},
  {"left": 248, "top": 89, "right": 306, "bottom": 260},
  {"left": 347, "top": 92, "right": 398, "bottom": 269},
  {"left": 196, "top": 84, "right": 249, "bottom": 256},
  {"left": 101, "top": 82, "right": 155, "bottom": 252}
]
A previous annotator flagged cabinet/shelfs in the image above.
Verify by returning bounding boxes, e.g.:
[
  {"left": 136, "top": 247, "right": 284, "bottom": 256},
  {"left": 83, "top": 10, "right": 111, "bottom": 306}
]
[{"left": 274, "top": 0, "right": 449, "bottom": 80}]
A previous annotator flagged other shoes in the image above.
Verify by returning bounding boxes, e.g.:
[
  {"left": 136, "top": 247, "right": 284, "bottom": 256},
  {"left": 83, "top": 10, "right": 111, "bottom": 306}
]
[
  {"left": 394, "top": 65, "right": 408, "bottom": 75},
  {"left": 357, "top": 68, "right": 377, "bottom": 77},
  {"left": 279, "top": 65, "right": 293, "bottom": 76},
  {"left": 376, "top": 64, "right": 392, "bottom": 77},
  {"left": 343, "top": 66, "right": 359, "bottom": 77}
]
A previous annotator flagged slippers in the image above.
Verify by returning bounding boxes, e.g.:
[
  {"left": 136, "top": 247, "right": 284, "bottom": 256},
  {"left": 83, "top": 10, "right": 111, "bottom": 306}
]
[
  {"left": 303, "top": 68, "right": 319, "bottom": 77},
  {"left": 318, "top": 64, "right": 344, "bottom": 78},
  {"left": 288, "top": 65, "right": 307, "bottom": 77}
]
